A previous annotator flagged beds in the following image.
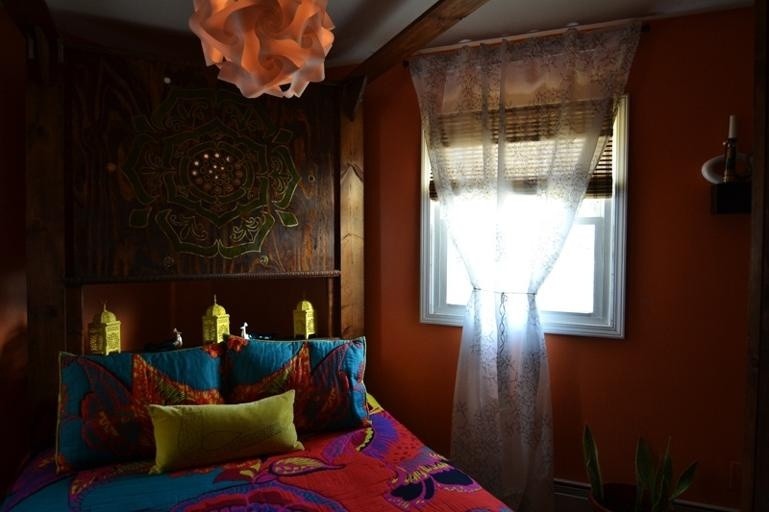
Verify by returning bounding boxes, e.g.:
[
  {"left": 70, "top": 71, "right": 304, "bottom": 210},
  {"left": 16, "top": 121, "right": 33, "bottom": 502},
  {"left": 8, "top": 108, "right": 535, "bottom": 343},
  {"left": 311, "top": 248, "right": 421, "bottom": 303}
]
[{"left": 0, "top": 271, "right": 519, "bottom": 512}]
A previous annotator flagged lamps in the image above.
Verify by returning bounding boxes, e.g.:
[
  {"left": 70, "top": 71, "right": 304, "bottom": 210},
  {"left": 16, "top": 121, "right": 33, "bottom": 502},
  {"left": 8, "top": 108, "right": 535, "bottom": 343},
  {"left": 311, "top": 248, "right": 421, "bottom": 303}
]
[{"left": 189, "top": 1, "right": 336, "bottom": 100}]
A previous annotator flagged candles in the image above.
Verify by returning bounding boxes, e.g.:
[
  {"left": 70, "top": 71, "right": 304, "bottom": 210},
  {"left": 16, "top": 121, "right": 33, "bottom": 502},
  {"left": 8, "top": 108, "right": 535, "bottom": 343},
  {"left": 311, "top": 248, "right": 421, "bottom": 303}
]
[{"left": 727, "top": 112, "right": 738, "bottom": 139}]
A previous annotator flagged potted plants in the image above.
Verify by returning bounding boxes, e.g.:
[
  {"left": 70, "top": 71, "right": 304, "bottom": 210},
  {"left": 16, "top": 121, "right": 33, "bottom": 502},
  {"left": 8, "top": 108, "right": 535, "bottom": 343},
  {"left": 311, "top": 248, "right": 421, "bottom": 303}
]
[{"left": 583, "top": 424, "right": 698, "bottom": 512}]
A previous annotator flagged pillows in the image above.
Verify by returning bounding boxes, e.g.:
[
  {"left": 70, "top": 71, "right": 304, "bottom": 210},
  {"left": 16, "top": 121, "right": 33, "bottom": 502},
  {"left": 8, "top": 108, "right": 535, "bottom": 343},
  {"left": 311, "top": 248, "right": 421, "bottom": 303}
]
[
  {"left": 222, "top": 333, "right": 370, "bottom": 428},
  {"left": 144, "top": 389, "right": 305, "bottom": 475},
  {"left": 54, "top": 340, "right": 225, "bottom": 477}
]
